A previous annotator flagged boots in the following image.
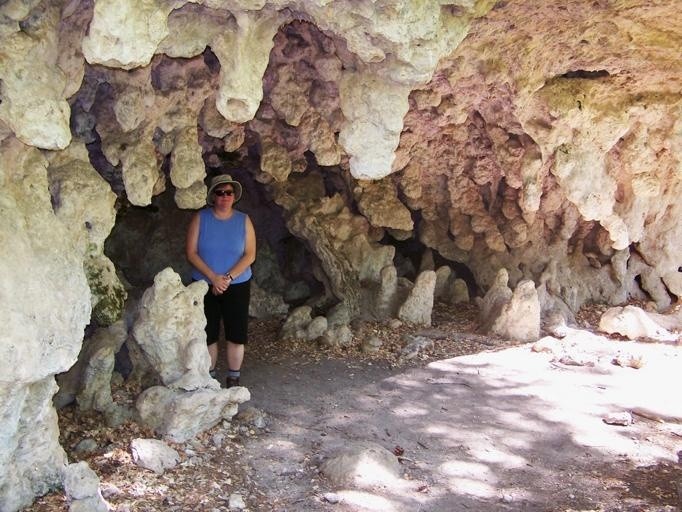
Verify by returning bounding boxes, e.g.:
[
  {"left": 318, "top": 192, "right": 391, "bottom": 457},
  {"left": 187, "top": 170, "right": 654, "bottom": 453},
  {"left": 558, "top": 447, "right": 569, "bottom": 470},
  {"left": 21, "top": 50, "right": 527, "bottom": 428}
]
[{"left": 226, "top": 376, "right": 239, "bottom": 388}]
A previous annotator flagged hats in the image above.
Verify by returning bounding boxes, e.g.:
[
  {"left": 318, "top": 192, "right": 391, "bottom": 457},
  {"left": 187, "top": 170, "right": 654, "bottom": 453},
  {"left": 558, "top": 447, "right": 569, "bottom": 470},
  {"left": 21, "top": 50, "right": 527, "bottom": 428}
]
[{"left": 207, "top": 174, "right": 242, "bottom": 206}]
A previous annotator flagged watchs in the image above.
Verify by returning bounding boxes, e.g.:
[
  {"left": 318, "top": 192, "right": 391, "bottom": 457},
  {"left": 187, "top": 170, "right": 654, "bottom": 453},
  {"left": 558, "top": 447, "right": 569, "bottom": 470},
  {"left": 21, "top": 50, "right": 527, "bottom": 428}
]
[{"left": 224, "top": 272, "right": 234, "bottom": 281}]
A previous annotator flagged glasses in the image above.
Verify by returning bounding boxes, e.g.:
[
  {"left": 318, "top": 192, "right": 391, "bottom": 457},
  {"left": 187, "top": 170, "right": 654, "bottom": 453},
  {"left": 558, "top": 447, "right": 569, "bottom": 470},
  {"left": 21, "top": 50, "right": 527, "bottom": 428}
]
[{"left": 215, "top": 190, "right": 234, "bottom": 195}]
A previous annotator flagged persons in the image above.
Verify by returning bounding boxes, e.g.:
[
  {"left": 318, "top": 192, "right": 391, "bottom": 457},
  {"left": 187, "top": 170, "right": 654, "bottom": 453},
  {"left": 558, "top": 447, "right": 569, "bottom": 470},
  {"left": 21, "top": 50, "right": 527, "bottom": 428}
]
[{"left": 185, "top": 174, "right": 257, "bottom": 389}]
